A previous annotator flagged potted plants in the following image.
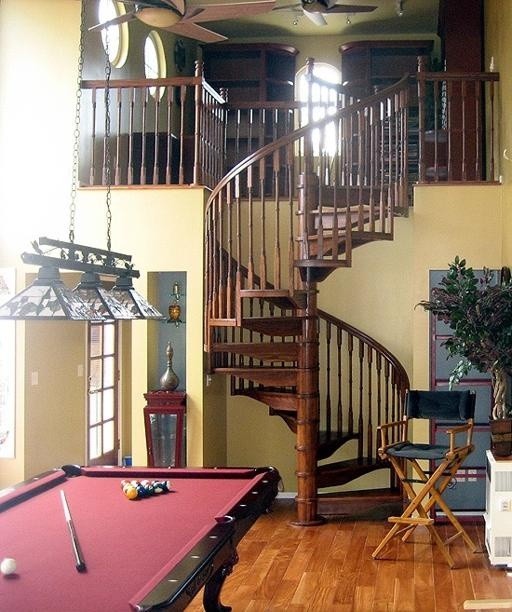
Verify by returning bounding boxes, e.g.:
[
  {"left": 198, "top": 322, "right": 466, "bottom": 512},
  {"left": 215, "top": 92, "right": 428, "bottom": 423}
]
[
  {"left": 414, "top": 255, "right": 512, "bottom": 457},
  {"left": 420, "top": 50, "right": 449, "bottom": 181}
]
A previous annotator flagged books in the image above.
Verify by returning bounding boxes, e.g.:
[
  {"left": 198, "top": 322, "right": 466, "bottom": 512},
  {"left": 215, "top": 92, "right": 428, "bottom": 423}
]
[{"left": 379, "top": 116, "right": 419, "bottom": 181}]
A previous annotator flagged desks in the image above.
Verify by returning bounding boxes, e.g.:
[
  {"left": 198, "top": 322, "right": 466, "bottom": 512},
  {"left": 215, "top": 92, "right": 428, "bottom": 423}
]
[
  {"left": 143, "top": 390, "right": 186, "bottom": 467},
  {"left": 0, "top": 464, "right": 280, "bottom": 612}
]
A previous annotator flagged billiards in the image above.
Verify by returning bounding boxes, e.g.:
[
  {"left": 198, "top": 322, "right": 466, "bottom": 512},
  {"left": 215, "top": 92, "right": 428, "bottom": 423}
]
[
  {"left": 0, "top": 558, "right": 17, "bottom": 577},
  {"left": 119, "top": 478, "right": 173, "bottom": 500}
]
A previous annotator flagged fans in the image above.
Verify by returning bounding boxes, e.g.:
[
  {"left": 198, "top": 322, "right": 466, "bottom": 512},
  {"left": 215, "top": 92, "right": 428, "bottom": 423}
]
[
  {"left": 274, "top": 0, "right": 378, "bottom": 27},
  {"left": 86, "top": 0, "right": 277, "bottom": 43}
]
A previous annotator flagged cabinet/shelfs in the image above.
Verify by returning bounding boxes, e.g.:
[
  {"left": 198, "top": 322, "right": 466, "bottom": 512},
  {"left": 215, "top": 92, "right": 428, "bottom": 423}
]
[
  {"left": 338, "top": 40, "right": 435, "bottom": 187},
  {"left": 199, "top": 43, "right": 300, "bottom": 197},
  {"left": 483, "top": 449, "right": 512, "bottom": 566}
]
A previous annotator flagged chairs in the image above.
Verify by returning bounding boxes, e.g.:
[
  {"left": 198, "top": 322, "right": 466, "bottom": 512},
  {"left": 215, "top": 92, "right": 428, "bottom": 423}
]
[{"left": 370, "top": 388, "right": 478, "bottom": 567}]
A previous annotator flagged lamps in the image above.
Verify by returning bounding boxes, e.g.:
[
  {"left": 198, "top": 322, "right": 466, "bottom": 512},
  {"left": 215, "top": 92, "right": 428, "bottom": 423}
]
[
  {"left": 168, "top": 282, "right": 185, "bottom": 327},
  {"left": 0, "top": 1, "right": 168, "bottom": 325}
]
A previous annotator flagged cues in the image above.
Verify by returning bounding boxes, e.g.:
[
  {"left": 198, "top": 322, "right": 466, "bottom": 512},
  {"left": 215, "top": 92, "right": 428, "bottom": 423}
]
[{"left": 60, "top": 489, "right": 86, "bottom": 571}]
